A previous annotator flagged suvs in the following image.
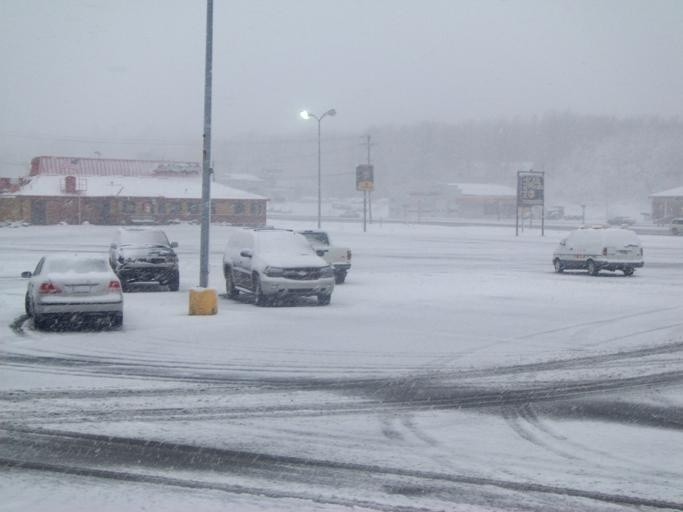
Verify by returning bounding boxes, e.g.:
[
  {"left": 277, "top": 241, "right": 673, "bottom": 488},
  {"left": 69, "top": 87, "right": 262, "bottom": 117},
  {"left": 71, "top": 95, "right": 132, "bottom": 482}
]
[
  {"left": 220, "top": 221, "right": 339, "bottom": 308},
  {"left": 107, "top": 223, "right": 181, "bottom": 293}
]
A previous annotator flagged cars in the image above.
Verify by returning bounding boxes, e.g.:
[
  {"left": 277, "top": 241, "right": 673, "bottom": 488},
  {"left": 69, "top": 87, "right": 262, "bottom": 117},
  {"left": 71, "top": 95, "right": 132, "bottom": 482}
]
[{"left": 18, "top": 251, "right": 125, "bottom": 329}]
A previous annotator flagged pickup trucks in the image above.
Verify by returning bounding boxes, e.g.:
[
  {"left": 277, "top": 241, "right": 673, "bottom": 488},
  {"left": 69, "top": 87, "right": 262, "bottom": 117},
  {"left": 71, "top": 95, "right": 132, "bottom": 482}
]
[{"left": 299, "top": 226, "right": 352, "bottom": 283}]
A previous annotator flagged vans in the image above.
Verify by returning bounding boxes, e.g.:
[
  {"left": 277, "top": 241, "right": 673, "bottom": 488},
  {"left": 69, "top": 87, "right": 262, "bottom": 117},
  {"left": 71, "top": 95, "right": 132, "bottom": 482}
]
[
  {"left": 668, "top": 216, "right": 682, "bottom": 236},
  {"left": 546, "top": 224, "right": 645, "bottom": 277}
]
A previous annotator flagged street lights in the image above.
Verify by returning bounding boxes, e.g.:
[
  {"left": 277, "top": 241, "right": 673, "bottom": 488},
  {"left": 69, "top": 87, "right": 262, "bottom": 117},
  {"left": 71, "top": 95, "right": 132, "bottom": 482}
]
[
  {"left": 296, "top": 108, "right": 340, "bottom": 230},
  {"left": 579, "top": 203, "right": 585, "bottom": 224}
]
